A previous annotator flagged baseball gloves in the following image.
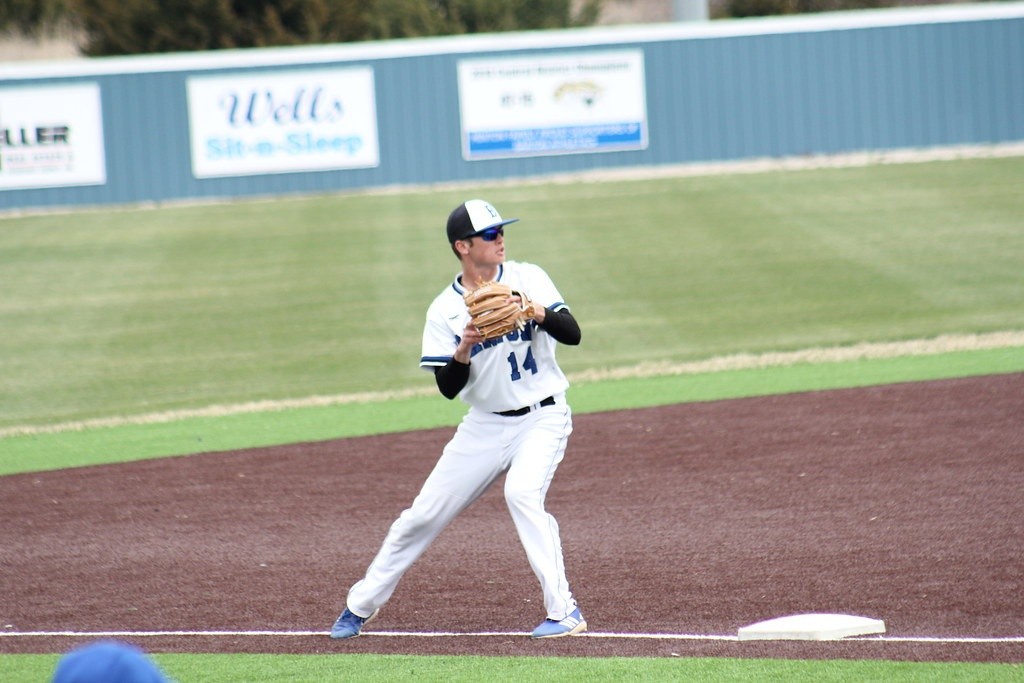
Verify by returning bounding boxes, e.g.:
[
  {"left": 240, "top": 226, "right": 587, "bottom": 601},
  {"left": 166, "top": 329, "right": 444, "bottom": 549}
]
[{"left": 462, "top": 275, "right": 536, "bottom": 342}]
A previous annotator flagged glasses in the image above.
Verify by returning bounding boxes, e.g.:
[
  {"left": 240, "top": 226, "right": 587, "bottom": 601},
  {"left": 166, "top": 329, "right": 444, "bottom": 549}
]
[{"left": 470, "top": 230, "right": 505, "bottom": 242}]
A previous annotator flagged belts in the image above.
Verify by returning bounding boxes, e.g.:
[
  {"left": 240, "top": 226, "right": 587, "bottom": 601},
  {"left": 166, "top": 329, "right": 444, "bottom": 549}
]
[{"left": 492, "top": 397, "right": 555, "bottom": 416}]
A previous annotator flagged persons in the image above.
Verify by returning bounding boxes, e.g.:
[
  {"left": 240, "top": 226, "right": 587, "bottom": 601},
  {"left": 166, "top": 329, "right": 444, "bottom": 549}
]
[{"left": 330, "top": 199, "right": 588, "bottom": 638}]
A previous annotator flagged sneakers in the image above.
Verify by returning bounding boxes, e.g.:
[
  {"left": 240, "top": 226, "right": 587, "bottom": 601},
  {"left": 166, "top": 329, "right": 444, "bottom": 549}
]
[
  {"left": 330, "top": 606, "right": 379, "bottom": 640},
  {"left": 529, "top": 609, "right": 588, "bottom": 639}
]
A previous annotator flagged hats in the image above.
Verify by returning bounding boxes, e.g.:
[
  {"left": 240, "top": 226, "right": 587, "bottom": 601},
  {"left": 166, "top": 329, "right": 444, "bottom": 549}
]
[{"left": 447, "top": 199, "right": 519, "bottom": 241}]
